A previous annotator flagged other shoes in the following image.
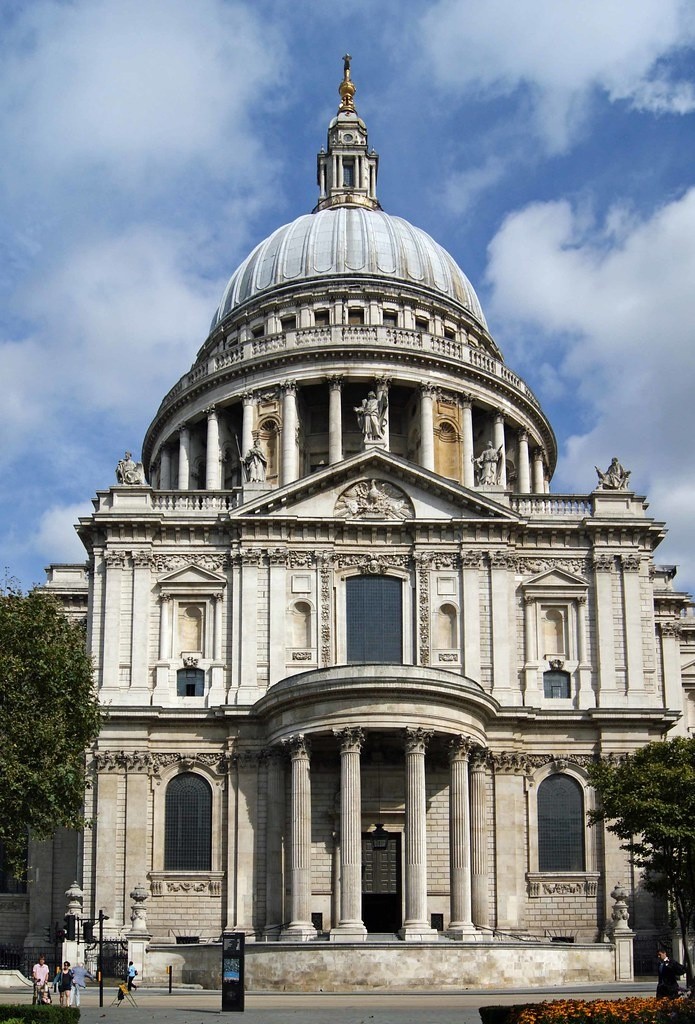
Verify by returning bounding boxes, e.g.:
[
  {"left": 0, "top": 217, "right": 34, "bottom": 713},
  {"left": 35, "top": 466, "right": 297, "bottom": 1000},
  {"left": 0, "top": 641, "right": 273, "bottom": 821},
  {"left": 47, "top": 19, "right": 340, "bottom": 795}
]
[{"left": 135, "top": 987, "right": 137, "bottom": 991}]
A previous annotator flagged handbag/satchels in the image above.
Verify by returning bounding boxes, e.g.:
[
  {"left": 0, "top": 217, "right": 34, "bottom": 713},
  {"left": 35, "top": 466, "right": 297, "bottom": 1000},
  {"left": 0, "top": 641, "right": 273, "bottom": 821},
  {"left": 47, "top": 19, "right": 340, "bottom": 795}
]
[{"left": 135, "top": 971, "right": 138, "bottom": 975}]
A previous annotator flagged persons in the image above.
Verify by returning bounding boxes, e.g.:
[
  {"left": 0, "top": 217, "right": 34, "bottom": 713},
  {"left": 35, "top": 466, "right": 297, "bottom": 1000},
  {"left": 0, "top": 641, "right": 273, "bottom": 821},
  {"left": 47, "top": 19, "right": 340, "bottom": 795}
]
[
  {"left": 471, "top": 441, "right": 503, "bottom": 486},
  {"left": 656, "top": 949, "right": 687, "bottom": 1001},
  {"left": 33, "top": 955, "right": 49, "bottom": 1004},
  {"left": 115, "top": 451, "right": 145, "bottom": 486},
  {"left": 603, "top": 458, "right": 630, "bottom": 489},
  {"left": 240, "top": 440, "right": 267, "bottom": 482},
  {"left": 127, "top": 961, "right": 137, "bottom": 991},
  {"left": 51, "top": 961, "right": 74, "bottom": 1006},
  {"left": 354, "top": 391, "right": 387, "bottom": 440},
  {"left": 69, "top": 963, "right": 95, "bottom": 1007}
]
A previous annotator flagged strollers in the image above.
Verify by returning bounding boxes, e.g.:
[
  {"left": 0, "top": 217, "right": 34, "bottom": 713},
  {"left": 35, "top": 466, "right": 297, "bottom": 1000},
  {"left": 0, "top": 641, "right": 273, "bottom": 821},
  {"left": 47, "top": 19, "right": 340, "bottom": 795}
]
[{"left": 35, "top": 981, "right": 53, "bottom": 1004}]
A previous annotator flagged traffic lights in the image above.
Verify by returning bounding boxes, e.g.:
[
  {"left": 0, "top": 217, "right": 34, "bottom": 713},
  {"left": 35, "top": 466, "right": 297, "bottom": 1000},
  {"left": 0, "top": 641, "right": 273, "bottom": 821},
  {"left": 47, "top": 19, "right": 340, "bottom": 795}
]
[
  {"left": 43, "top": 922, "right": 57, "bottom": 944},
  {"left": 58, "top": 914, "right": 76, "bottom": 942}
]
